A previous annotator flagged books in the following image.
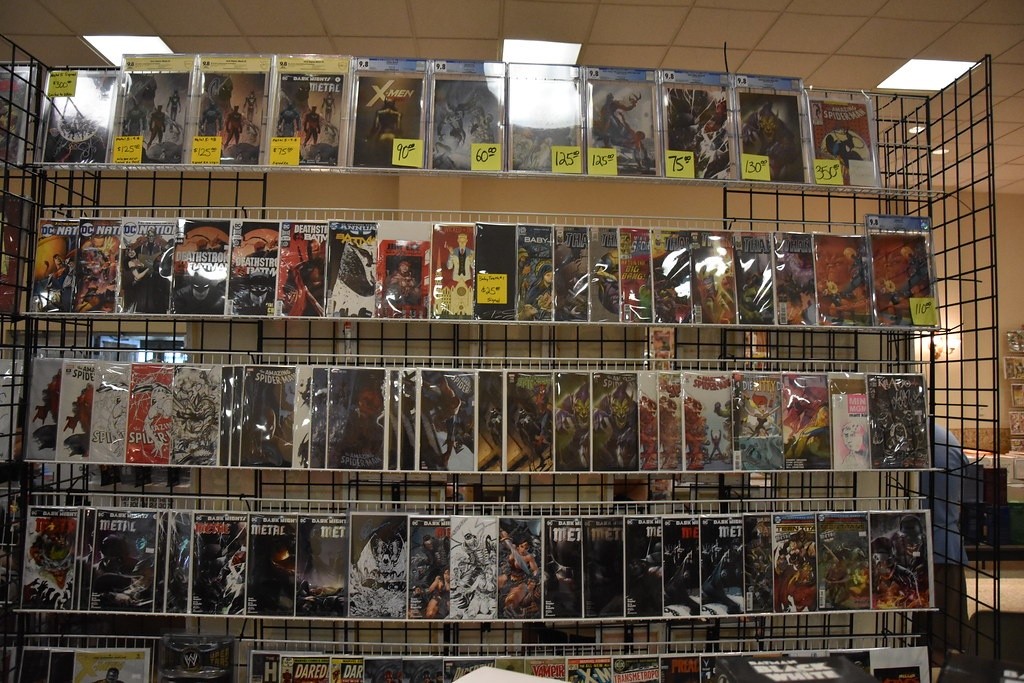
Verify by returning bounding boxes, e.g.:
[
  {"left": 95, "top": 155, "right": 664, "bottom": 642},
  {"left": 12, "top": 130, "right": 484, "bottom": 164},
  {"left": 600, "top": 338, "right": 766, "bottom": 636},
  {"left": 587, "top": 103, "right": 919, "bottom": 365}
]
[
  {"left": 24, "top": 354, "right": 932, "bottom": 473},
  {"left": 1, "top": 641, "right": 929, "bottom": 683},
  {"left": 18, "top": 509, "right": 937, "bottom": 624},
  {"left": 0, "top": 52, "right": 883, "bottom": 191},
  {"left": 28, "top": 219, "right": 939, "bottom": 328}
]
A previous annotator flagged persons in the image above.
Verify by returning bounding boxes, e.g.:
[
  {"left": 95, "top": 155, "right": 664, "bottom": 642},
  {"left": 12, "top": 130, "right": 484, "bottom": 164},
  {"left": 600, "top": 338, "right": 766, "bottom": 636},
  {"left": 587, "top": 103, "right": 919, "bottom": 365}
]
[{"left": 922, "top": 425, "right": 970, "bottom": 668}]
